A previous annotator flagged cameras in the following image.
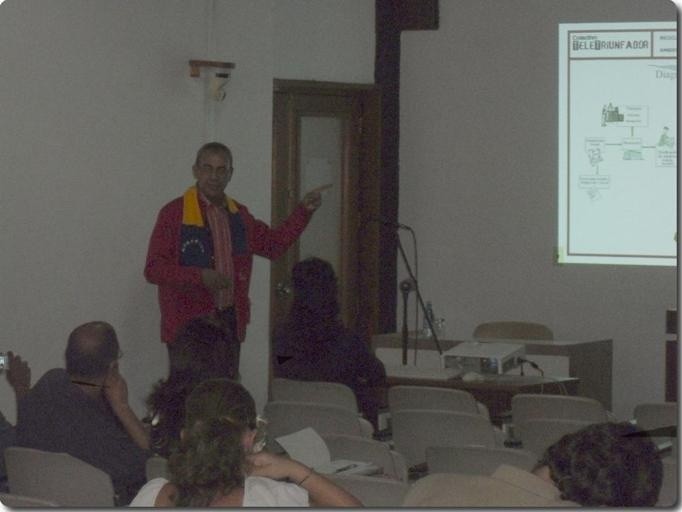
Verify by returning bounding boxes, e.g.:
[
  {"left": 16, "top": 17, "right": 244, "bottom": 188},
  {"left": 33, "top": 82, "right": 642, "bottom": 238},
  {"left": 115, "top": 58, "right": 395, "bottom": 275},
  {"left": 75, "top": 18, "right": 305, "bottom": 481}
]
[{"left": 0, "top": 352, "right": 10, "bottom": 370}]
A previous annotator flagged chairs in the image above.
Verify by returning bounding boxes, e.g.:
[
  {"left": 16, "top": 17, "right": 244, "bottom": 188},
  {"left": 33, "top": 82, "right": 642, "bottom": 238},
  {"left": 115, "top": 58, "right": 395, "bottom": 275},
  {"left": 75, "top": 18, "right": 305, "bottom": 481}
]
[
  {"left": 146, "top": 457, "right": 254, "bottom": 488},
  {"left": 1, "top": 445, "right": 126, "bottom": 508},
  {"left": 473, "top": 321, "right": 553, "bottom": 340},
  {"left": 259, "top": 380, "right": 678, "bottom": 508}
]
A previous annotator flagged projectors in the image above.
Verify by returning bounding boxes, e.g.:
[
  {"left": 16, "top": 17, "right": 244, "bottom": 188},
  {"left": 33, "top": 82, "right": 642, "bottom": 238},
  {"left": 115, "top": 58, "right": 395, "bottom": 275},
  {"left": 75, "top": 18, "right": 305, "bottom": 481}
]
[{"left": 440, "top": 342, "right": 526, "bottom": 377}]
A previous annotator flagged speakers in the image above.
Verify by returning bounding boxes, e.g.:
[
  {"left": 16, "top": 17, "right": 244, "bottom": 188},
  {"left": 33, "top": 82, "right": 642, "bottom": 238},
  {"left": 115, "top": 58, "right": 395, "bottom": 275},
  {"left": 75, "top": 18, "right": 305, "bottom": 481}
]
[{"left": 375, "top": 0, "right": 439, "bottom": 32}]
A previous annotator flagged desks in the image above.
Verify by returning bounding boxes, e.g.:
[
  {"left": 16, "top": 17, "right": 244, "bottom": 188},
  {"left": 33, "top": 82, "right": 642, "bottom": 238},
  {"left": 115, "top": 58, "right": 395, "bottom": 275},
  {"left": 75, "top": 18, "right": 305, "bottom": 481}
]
[{"left": 375, "top": 348, "right": 579, "bottom": 413}]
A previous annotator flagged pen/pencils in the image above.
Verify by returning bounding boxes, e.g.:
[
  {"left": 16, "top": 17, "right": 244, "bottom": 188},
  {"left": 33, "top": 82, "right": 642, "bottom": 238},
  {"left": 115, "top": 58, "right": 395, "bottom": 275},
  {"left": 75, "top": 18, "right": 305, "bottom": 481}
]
[{"left": 336, "top": 464, "right": 357, "bottom": 473}]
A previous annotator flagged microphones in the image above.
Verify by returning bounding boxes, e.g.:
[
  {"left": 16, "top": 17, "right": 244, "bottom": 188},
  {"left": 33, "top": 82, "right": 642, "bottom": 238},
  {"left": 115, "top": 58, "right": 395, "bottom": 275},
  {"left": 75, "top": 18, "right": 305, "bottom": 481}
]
[{"left": 365, "top": 213, "right": 412, "bottom": 232}]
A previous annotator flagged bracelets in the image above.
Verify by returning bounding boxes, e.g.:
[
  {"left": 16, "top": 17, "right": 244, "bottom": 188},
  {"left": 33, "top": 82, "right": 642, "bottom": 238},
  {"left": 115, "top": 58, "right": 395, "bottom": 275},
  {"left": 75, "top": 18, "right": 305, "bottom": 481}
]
[{"left": 298, "top": 467, "right": 314, "bottom": 485}]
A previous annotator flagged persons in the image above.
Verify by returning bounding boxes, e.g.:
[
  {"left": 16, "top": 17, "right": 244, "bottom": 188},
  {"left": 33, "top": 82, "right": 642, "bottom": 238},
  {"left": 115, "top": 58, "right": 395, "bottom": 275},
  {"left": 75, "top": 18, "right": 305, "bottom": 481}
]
[
  {"left": 270, "top": 257, "right": 387, "bottom": 427},
  {"left": 1, "top": 350, "right": 32, "bottom": 476},
  {"left": 144, "top": 142, "right": 333, "bottom": 381},
  {"left": 141, "top": 334, "right": 230, "bottom": 457},
  {"left": 130, "top": 378, "right": 363, "bottom": 508},
  {"left": 24, "top": 322, "right": 151, "bottom": 504},
  {"left": 401, "top": 421, "right": 663, "bottom": 508}
]
